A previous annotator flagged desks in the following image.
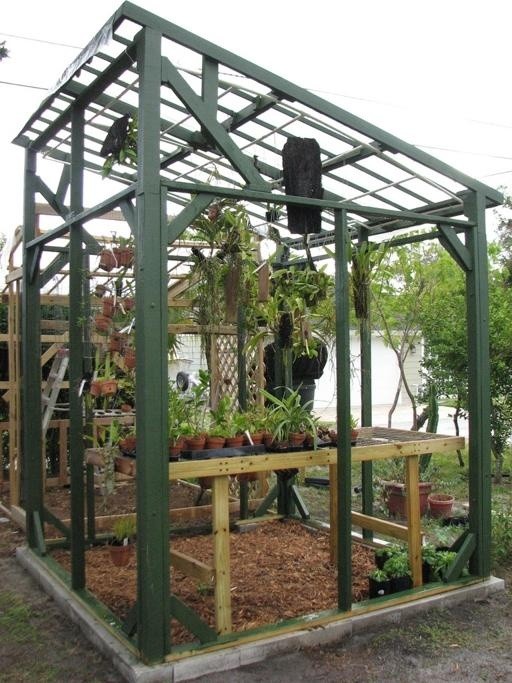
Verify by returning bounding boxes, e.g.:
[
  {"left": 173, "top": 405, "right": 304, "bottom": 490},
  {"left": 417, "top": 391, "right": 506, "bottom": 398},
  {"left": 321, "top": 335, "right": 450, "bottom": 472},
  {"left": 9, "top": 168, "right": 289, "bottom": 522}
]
[{"left": 81, "top": 426, "right": 467, "bottom": 625}]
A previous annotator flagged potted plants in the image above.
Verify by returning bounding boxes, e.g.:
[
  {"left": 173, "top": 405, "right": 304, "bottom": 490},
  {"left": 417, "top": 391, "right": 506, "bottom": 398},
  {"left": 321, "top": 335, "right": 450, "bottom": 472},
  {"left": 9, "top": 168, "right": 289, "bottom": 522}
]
[{"left": 80, "top": 162, "right": 455, "bottom": 594}]
